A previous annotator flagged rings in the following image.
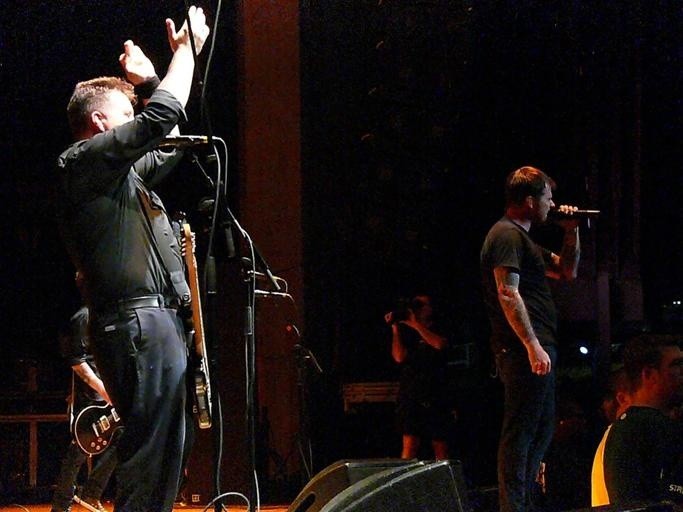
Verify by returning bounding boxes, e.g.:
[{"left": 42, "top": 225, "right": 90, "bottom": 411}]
[{"left": 538, "top": 367, "right": 543, "bottom": 370}]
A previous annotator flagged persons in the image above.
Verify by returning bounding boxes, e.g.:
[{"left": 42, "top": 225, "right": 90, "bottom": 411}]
[
  {"left": 51, "top": 270, "right": 124, "bottom": 512},
  {"left": 56, "top": 4, "right": 212, "bottom": 512},
  {"left": 479, "top": 165, "right": 582, "bottom": 512},
  {"left": 590, "top": 333, "right": 683, "bottom": 512},
  {"left": 384, "top": 296, "right": 452, "bottom": 459}
]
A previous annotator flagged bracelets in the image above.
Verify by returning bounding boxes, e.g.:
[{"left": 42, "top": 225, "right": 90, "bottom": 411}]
[{"left": 134, "top": 77, "right": 160, "bottom": 100}]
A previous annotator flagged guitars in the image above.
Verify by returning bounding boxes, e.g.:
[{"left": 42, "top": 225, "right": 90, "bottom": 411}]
[
  {"left": 72, "top": 400, "right": 124, "bottom": 457},
  {"left": 180, "top": 223, "right": 212, "bottom": 428}
]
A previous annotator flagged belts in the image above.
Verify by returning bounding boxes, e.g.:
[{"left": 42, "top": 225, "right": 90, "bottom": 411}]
[{"left": 92, "top": 296, "right": 160, "bottom": 315}]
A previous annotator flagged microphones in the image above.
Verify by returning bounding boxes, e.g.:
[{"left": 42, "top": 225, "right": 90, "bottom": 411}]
[
  {"left": 149, "top": 134, "right": 221, "bottom": 148},
  {"left": 552, "top": 208, "right": 601, "bottom": 218}
]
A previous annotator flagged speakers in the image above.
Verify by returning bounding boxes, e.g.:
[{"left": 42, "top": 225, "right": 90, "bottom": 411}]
[{"left": 285, "top": 458, "right": 464, "bottom": 512}]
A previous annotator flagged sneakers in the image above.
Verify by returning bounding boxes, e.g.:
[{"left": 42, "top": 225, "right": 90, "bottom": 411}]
[{"left": 74, "top": 494, "right": 105, "bottom": 512}]
